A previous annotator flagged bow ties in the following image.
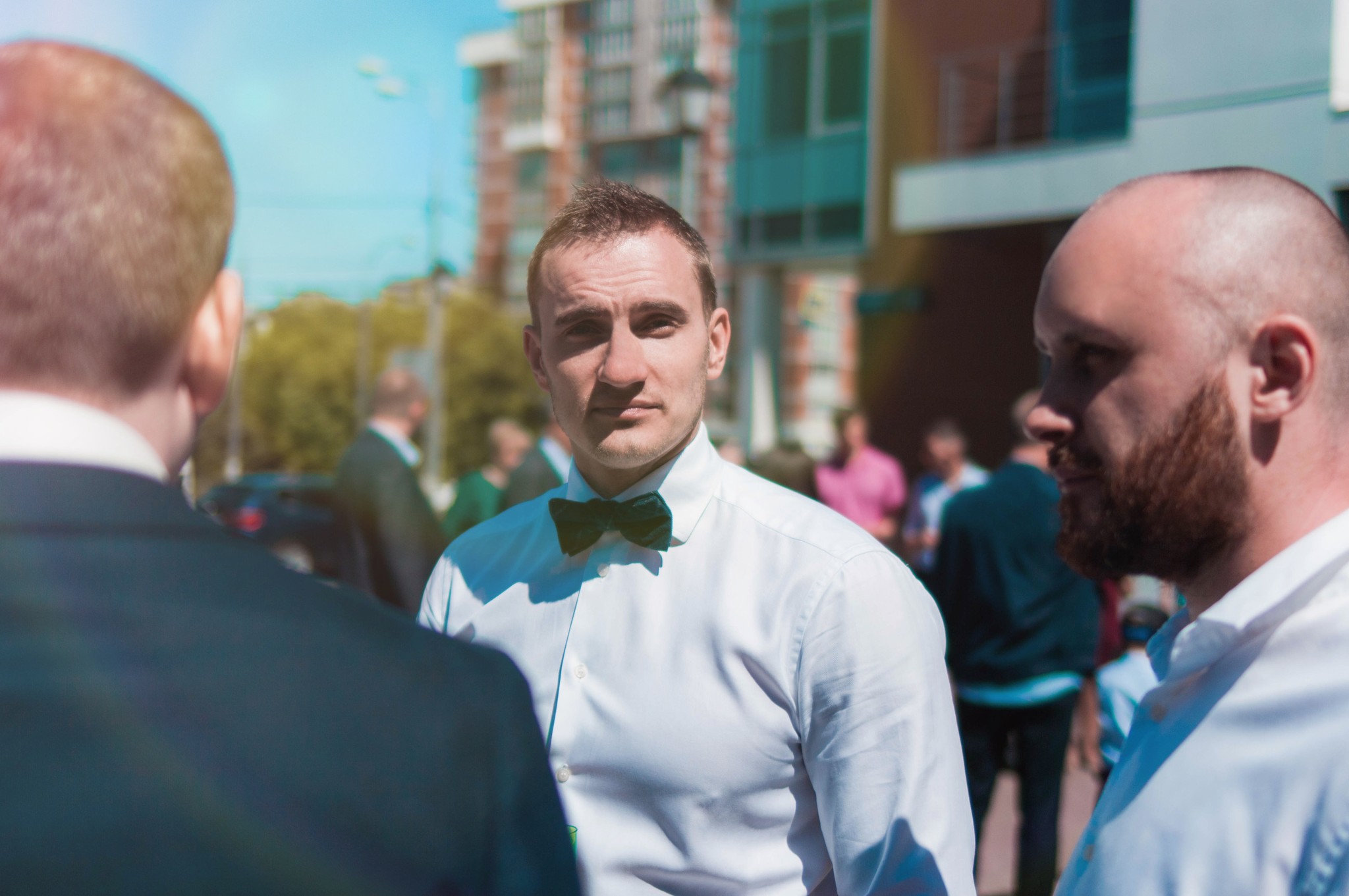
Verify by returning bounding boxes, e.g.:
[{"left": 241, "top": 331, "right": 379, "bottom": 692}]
[{"left": 548, "top": 492, "right": 673, "bottom": 557}]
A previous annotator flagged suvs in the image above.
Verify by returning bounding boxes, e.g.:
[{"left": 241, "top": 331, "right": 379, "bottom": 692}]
[{"left": 195, "top": 472, "right": 360, "bottom": 583}]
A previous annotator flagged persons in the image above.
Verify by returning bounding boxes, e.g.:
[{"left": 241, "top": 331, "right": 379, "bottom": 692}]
[
  {"left": 913, "top": 385, "right": 1102, "bottom": 896},
  {"left": 0, "top": 37, "right": 593, "bottom": 895},
  {"left": 1023, "top": 164, "right": 1349, "bottom": 895},
  {"left": 1073, "top": 573, "right": 1192, "bottom": 796},
  {"left": 390, "top": 181, "right": 981, "bottom": 896},
  {"left": 330, "top": 365, "right": 454, "bottom": 630},
  {"left": 452, "top": 394, "right": 984, "bottom": 607}
]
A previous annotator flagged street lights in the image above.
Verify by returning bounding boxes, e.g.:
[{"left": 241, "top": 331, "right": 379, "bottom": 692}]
[{"left": 360, "top": 56, "right": 442, "bottom": 474}]
